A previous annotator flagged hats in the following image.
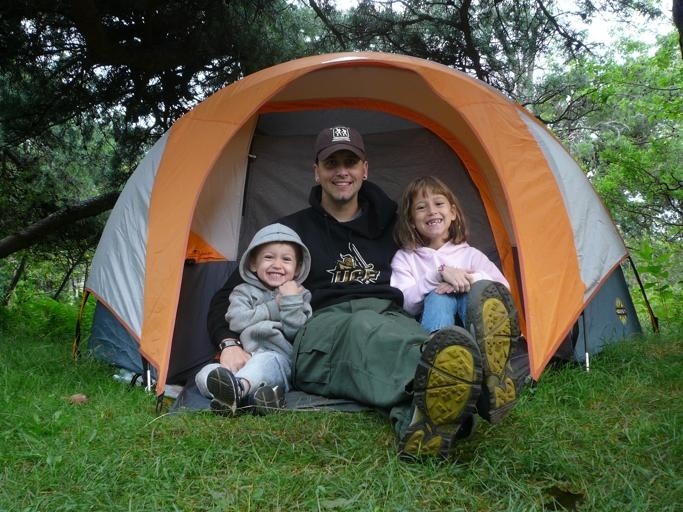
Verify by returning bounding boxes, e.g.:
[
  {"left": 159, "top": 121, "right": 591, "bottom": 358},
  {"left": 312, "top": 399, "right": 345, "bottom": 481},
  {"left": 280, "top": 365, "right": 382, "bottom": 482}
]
[{"left": 315, "top": 125, "right": 366, "bottom": 162}]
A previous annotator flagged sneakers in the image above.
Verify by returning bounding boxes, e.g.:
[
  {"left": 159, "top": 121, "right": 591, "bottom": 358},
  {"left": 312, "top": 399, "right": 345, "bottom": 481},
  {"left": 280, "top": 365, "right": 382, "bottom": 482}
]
[
  {"left": 248, "top": 384, "right": 286, "bottom": 414},
  {"left": 206, "top": 368, "right": 240, "bottom": 418},
  {"left": 397, "top": 279, "right": 519, "bottom": 466}
]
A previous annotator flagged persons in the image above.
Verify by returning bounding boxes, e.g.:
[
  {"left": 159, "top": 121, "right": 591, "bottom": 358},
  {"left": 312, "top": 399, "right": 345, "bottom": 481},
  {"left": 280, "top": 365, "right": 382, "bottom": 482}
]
[
  {"left": 387, "top": 174, "right": 511, "bottom": 334},
  {"left": 194, "top": 222, "right": 313, "bottom": 419},
  {"left": 205, "top": 126, "right": 521, "bottom": 471}
]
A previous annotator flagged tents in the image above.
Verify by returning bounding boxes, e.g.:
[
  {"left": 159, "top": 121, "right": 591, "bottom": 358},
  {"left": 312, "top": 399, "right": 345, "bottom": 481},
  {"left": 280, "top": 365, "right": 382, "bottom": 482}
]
[{"left": 68, "top": 52, "right": 662, "bottom": 424}]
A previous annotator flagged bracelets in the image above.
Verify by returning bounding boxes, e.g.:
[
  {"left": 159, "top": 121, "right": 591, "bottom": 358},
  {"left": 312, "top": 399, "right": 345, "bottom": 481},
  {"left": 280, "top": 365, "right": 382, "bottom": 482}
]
[
  {"left": 217, "top": 339, "right": 241, "bottom": 351},
  {"left": 437, "top": 264, "right": 445, "bottom": 282}
]
